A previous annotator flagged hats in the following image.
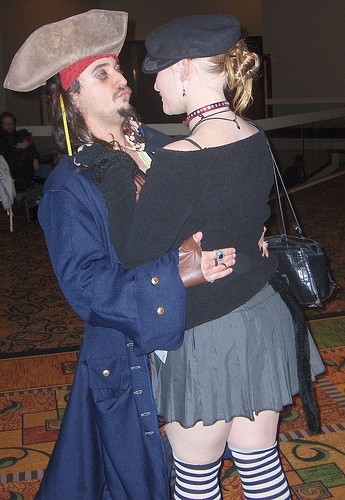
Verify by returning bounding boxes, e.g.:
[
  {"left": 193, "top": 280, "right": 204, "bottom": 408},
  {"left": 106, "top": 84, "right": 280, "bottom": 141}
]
[
  {"left": 3, "top": 9, "right": 128, "bottom": 92},
  {"left": 142, "top": 14, "right": 242, "bottom": 76}
]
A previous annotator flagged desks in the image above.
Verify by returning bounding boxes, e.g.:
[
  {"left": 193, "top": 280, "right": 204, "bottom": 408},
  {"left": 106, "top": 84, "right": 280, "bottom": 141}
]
[{"left": 0, "top": 155, "right": 16, "bottom": 233}]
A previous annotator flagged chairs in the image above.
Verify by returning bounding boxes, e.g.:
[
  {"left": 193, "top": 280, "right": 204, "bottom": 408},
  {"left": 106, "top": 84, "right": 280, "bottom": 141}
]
[{"left": 16, "top": 157, "right": 57, "bottom": 223}]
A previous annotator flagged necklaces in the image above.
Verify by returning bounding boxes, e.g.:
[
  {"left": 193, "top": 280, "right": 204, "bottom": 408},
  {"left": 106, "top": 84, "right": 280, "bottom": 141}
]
[{"left": 184, "top": 101, "right": 240, "bottom": 136}]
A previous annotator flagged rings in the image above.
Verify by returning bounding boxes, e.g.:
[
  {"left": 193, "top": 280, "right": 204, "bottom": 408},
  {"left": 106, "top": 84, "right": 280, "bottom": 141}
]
[
  {"left": 221, "top": 263, "right": 227, "bottom": 271},
  {"left": 215, "top": 259, "right": 218, "bottom": 266},
  {"left": 216, "top": 251, "right": 223, "bottom": 260}
]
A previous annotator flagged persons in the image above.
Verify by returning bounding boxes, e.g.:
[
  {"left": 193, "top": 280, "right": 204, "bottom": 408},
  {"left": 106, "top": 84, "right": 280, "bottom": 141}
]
[
  {"left": 74, "top": 15, "right": 325, "bottom": 500},
  {"left": 0, "top": 112, "right": 48, "bottom": 220},
  {"left": 2, "top": 10, "right": 269, "bottom": 500}
]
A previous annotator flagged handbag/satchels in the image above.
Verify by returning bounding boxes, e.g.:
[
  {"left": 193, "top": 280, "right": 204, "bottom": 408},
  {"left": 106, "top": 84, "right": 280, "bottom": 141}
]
[{"left": 247, "top": 120, "right": 338, "bottom": 308}]
